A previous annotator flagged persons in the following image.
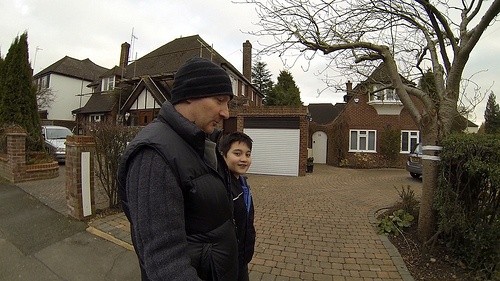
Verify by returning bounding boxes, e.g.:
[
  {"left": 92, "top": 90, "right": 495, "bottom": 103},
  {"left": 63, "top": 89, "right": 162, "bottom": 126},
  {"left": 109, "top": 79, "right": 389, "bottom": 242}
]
[
  {"left": 118, "top": 58, "right": 239, "bottom": 281},
  {"left": 216, "top": 131, "right": 256, "bottom": 281}
]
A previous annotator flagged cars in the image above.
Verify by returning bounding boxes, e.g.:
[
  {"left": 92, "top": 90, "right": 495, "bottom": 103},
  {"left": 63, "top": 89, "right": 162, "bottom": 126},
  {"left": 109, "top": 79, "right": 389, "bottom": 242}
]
[
  {"left": 406, "top": 143, "right": 422, "bottom": 180},
  {"left": 42, "top": 126, "right": 75, "bottom": 164}
]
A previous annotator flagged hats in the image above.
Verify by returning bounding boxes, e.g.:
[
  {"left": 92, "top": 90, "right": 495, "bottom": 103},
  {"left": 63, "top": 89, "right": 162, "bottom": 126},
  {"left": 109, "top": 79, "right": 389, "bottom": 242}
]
[{"left": 171, "top": 57, "right": 233, "bottom": 104}]
